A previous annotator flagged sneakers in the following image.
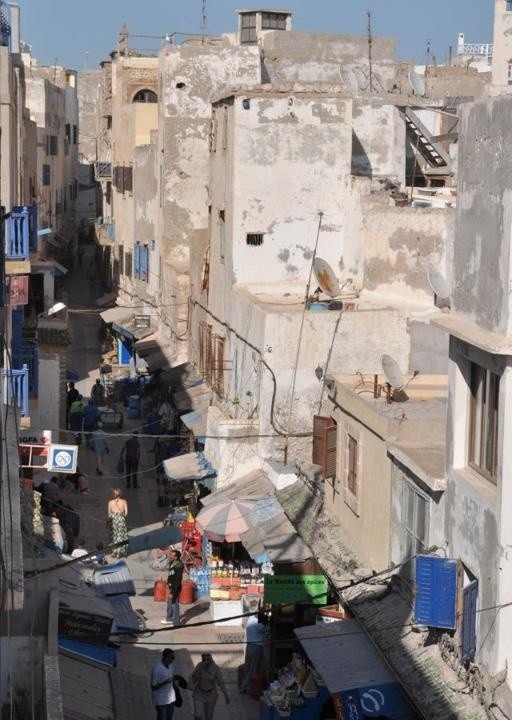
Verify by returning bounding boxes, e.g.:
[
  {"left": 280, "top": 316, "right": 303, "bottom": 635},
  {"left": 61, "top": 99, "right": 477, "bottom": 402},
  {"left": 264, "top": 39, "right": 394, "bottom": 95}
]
[{"left": 160, "top": 619, "right": 175, "bottom": 627}]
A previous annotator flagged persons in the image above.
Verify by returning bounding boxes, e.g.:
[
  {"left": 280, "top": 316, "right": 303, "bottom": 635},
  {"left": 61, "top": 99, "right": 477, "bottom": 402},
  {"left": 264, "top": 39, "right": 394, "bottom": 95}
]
[
  {"left": 76, "top": 246, "right": 83, "bottom": 268},
  {"left": 239, "top": 608, "right": 270, "bottom": 694},
  {"left": 71, "top": 540, "right": 90, "bottom": 562},
  {"left": 87, "top": 261, "right": 96, "bottom": 296},
  {"left": 160, "top": 550, "right": 184, "bottom": 628},
  {"left": 151, "top": 649, "right": 179, "bottom": 720},
  {"left": 43, "top": 379, "right": 140, "bottom": 508},
  {"left": 108, "top": 487, "right": 129, "bottom": 559},
  {"left": 188, "top": 652, "right": 229, "bottom": 719},
  {"left": 91, "top": 542, "right": 109, "bottom": 565}
]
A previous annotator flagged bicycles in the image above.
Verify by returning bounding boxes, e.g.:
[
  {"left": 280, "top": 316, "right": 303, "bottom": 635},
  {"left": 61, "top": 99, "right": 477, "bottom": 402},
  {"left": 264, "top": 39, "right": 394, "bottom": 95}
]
[{"left": 131, "top": 608, "right": 146, "bottom": 638}]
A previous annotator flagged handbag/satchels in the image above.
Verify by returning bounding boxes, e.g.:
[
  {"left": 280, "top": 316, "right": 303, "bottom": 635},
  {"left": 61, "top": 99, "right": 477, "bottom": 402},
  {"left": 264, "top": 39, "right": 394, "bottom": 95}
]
[
  {"left": 116, "top": 456, "right": 125, "bottom": 474},
  {"left": 187, "top": 675, "right": 199, "bottom": 691}
]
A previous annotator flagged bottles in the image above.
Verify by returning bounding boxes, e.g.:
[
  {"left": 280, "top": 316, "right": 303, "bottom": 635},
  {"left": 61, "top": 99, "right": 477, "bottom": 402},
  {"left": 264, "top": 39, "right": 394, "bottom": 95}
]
[{"left": 189, "top": 559, "right": 264, "bottom": 601}]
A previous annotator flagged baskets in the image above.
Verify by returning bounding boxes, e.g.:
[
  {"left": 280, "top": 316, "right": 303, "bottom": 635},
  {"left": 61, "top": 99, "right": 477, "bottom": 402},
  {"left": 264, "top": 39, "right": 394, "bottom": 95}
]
[{"left": 268, "top": 664, "right": 326, "bottom": 717}]
